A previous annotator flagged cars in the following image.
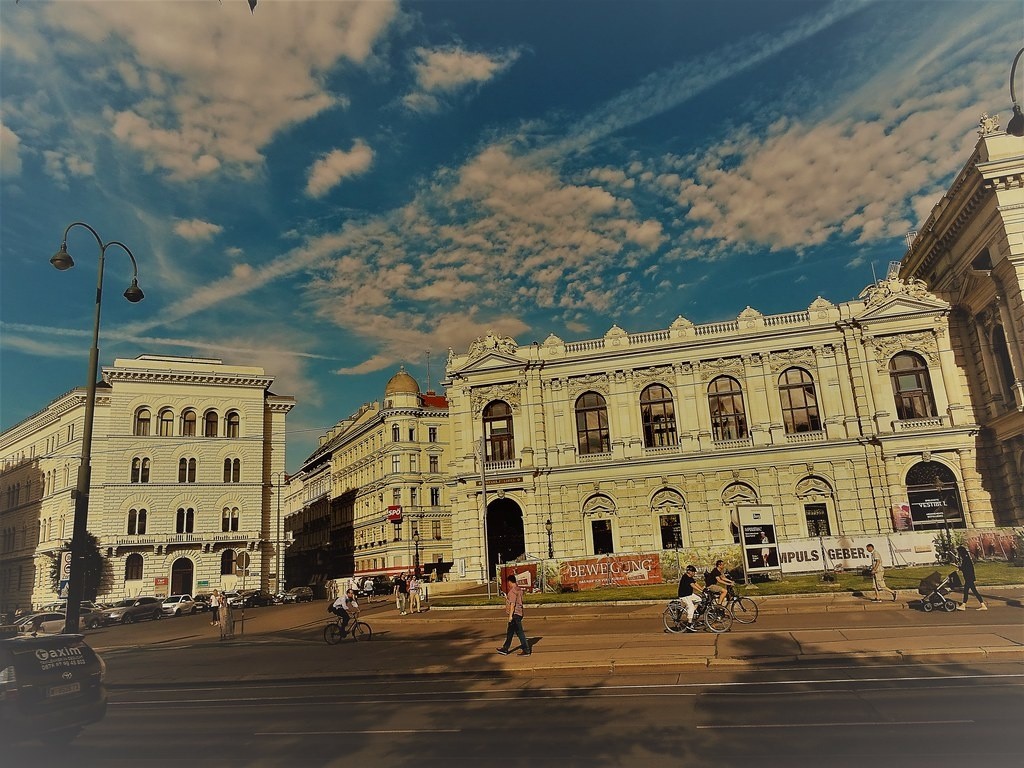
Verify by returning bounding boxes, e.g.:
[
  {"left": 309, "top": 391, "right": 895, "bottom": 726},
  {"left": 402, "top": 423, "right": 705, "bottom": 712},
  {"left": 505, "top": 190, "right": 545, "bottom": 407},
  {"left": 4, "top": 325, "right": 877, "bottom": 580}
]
[
  {"left": 195, "top": 589, "right": 271, "bottom": 611},
  {"left": 9, "top": 599, "right": 114, "bottom": 634},
  {"left": 104, "top": 597, "right": 162, "bottom": 624},
  {"left": 162, "top": 594, "right": 197, "bottom": 617}
]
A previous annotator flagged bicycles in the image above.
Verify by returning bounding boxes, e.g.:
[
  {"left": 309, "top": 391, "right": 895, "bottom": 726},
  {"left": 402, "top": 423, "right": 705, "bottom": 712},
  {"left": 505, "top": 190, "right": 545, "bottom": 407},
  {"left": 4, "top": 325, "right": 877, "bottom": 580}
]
[
  {"left": 324, "top": 611, "right": 371, "bottom": 645},
  {"left": 695, "top": 586, "right": 758, "bottom": 624},
  {"left": 662, "top": 589, "right": 733, "bottom": 634}
]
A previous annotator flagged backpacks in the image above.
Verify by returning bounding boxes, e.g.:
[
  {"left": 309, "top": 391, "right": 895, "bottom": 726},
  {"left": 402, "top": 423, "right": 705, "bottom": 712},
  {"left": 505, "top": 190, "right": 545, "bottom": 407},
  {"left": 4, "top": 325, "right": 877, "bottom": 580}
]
[{"left": 328, "top": 603, "right": 335, "bottom": 613}]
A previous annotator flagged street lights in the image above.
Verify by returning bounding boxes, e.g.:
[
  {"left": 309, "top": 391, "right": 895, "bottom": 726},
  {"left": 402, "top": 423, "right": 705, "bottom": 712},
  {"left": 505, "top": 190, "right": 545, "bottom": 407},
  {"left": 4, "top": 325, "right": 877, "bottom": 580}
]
[
  {"left": 934, "top": 476, "right": 952, "bottom": 550},
  {"left": 268, "top": 471, "right": 290, "bottom": 593},
  {"left": 50, "top": 222, "right": 144, "bottom": 634},
  {"left": 413, "top": 530, "right": 422, "bottom": 580},
  {"left": 545, "top": 519, "right": 554, "bottom": 559}
]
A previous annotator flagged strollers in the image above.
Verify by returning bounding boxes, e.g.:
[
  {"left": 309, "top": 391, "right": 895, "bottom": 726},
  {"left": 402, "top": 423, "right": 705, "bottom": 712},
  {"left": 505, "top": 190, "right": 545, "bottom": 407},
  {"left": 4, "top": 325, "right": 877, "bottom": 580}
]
[{"left": 919, "top": 569, "right": 961, "bottom": 612}]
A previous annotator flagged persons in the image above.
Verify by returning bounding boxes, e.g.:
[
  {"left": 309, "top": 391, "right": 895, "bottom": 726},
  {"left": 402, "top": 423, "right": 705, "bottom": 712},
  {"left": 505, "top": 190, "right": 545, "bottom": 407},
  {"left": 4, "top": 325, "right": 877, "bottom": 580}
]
[
  {"left": 326, "top": 569, "right": 443, "bottom": 615},
  {"left": 761, "top": 531, "right": 770, "bottom": 567},
  {"left": 7, "top": 607, "right": 24, "bottom": 626},
  {"left": 496, "top": 575, "right": 530, "bottom": 656},
  {"left": 866, "top": 544, "right": 898, "bottom": 602},
  {"left": 677, "top": 565, "right": 704, "bottom": 631},
  {"left": 950, "top": 545, "right": 988, "bottom": 611},
  {"left": 863, "top": 282, "right": 889, "bottom": 310},
  {"left": 905, "top": 278, "right": 950, "bottom": 306},
  {"left": 332, "top": 589, "right": 360, "bottom": 635},
  {"left": 706, "top": 560, "right": 735, "bottom": 611},
  {"left": 208, "top": 589, "right": 227, "bottom": 627}
]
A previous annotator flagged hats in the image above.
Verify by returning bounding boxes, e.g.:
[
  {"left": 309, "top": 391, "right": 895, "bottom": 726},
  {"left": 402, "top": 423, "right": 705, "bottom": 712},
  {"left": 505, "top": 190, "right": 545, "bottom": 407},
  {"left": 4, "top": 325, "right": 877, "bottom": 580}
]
[{"left": 687, "top": 565, "right": 697, "bottom": 572}]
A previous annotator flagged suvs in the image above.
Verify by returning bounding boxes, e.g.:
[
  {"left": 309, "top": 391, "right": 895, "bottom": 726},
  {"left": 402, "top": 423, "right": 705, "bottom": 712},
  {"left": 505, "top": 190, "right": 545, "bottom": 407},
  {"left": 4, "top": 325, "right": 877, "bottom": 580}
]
[
  {"left": 283, "top": 587, "right": 313, "bottom": 604},
  {"left": 358, "top": 575, "right": 398, "bottom": 595},
  {"left": 0, "top": 632, "right": 107, "bottom": 755}
]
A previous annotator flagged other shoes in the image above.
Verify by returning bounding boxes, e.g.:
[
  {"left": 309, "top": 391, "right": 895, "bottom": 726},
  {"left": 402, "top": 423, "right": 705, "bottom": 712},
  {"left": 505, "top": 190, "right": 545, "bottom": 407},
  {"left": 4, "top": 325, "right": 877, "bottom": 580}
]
[
  {"left": 694, "top": 610, "right": 699, "bottom": 615},
  {"left": 892, "top": 591, "right": 898, "bottom": 601},
  {"left": 686, "top": 622, "right": 697, "bottom": 632},
  {"left": 871, "top": 597, "right": 882, "bottom": 603},
  {"left": 496, "top": 648, "right": 508, "bottom": 655},
  {"left": 718, "top": 609, "right": 724, "bottom": 616},
  {"left": 517, "top": 651, "right": 530, "bottom": 656}
]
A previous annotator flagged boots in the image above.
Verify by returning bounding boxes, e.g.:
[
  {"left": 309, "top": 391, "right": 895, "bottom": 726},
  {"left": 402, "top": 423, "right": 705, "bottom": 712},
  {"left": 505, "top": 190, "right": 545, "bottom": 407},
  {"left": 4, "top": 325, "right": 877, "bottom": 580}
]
[
  {"left": 976, "top": 603, "right": 987, "bottom": 610},
  {"left": 956, "top": 603, "right": 965, "bottom": 611}
]
